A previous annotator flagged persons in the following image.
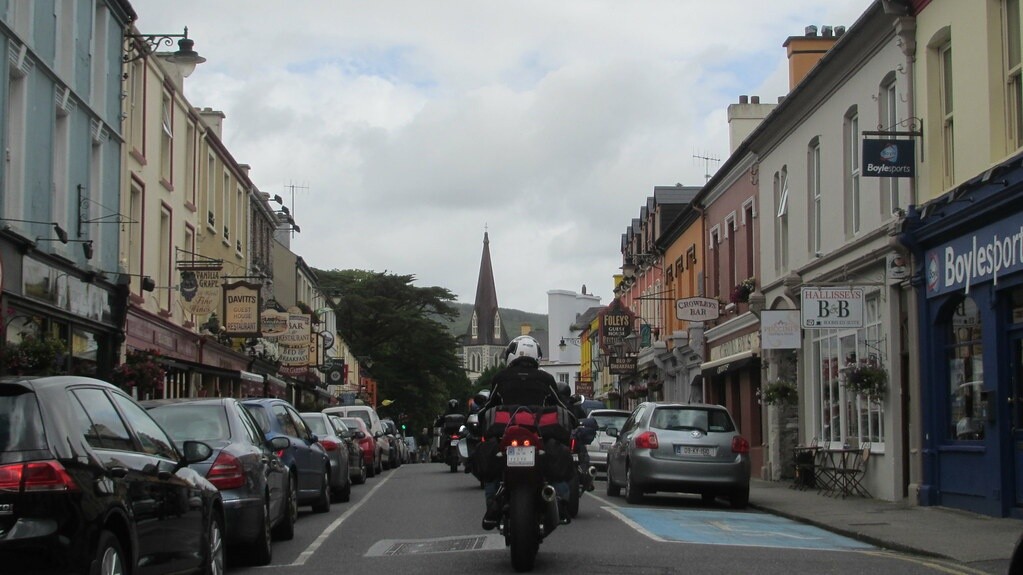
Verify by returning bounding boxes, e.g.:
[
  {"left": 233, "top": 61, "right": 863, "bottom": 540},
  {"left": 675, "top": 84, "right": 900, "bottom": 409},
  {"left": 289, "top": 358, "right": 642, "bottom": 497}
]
[
  {"left": 558, "top": 382, "right": 590, "bottom": 467},
  {"left": 472, "top": 335, "right": 580, "bottom": 531},
  {"left": 419, "top": 428, "right": 430, "bottom": 462},
  {"left": 435, "top": 390, "right": 490, "bottom": 474}
]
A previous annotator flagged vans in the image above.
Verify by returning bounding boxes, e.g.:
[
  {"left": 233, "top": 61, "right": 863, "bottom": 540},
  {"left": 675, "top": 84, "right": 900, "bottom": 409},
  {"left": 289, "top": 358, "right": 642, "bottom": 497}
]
[
  {"left": 321, "top": 405, "right": 391, "bottom": 468},
  {"left": 569, "top": 397, "right": 607, "bottom": 420}
]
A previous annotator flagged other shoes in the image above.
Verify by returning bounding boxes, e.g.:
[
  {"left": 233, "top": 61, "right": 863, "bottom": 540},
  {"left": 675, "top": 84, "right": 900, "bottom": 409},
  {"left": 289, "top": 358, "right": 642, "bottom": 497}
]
[{"left": 482, "top": 499, "right": 502, "bottom": 530}]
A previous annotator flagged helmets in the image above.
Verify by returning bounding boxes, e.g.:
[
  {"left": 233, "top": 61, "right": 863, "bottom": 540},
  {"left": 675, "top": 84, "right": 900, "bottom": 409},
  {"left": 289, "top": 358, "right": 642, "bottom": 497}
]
[
  {"left": 555, "top": 381, "right": 572, "bottom": 397},
  {"left": 449, "top": 399, "right": 458, "bottom": 409},
  {"left": 505, "top": 335, "right": 542, "bottom": 367},
  {"left": 476, "top": 389, "right": 491, "bottom": 405}
]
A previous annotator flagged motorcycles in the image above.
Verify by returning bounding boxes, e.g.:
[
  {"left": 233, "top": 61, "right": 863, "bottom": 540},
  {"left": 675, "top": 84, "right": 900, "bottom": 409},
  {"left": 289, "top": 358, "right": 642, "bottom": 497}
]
[{"left": 439, "top": 389, "right": 599, "bottom": 572}]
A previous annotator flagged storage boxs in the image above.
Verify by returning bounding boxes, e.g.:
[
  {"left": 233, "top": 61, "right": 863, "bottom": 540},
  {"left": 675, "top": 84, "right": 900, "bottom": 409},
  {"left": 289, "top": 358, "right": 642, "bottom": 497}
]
[{"left": 442, "top": 413, "right": 466, "bottom": 432}]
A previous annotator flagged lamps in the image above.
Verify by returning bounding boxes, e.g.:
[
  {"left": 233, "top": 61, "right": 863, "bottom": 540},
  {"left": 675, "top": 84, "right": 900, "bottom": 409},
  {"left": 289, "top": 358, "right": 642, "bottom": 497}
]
[
  {"left": 251, "top": 194, "right": 300, "bottom": 233},
  {"left": 559, "top": 335, "right": 584, "bottom": 351},
  {"left": 0, "top": 217, "right": 69, "bottom": 244},
  {"left": 591, "top": 316, "right": 660, "bottom": 372},
  {"left": 313, "top": 285, "right": 344, "bottom": 305},
  {"left": 121, "top": 25, "right": 206, "bottom": 78},
  {"left": 34, "top": 237, "right": 93, "bottom": 260},
  {"left": 619, "top": 251, "right": 665, "bottom": 278},
  {"left": 101, "top": 270, "right": 155, "bottom": 292}
]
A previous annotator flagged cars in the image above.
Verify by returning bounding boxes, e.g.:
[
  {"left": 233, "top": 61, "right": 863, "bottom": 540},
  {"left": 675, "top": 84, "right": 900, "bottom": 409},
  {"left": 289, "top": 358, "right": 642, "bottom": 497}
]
[
  {"left": 568, "top": 399, "right": 752, "bottom": 509},
  {"left": 0, "top": 375, "right": 226, "bottom": 575},
  {"left": 135, "top": 397, "right": 409, "bottom": 567}
]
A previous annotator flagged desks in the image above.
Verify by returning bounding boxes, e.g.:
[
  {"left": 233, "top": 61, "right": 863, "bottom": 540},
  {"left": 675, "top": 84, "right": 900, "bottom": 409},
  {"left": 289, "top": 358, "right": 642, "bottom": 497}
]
[
  {"left": 824, "top": 448, "right": 866, "bottom": 499},
  {"left": 788, "top": 446, "right": 824, "bottom": 492}
]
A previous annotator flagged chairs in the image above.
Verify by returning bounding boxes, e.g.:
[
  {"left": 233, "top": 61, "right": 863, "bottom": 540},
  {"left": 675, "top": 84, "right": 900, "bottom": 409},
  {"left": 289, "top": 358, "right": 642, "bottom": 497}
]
[{"left": 787, "top": 436, "right": 872, "bottom": 499}]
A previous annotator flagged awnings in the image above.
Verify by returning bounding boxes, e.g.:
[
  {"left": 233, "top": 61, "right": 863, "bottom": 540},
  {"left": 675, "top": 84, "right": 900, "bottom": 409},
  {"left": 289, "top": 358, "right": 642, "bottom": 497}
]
[
  {"left": 289, "top": 376, "right": 330, "bottom": 397},
  {"left": 134, "top": 347, "right": 286, "bottom": 389}
]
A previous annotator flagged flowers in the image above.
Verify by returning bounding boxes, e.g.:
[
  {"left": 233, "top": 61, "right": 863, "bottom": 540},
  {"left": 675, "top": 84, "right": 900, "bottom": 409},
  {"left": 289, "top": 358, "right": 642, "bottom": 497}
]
[
  {"left": 839, "top": 356, "right": 888, "bottom": 406},
  {"left": 755, "top": 377, "right": 798, "bottom": 413},
  {"left": 110, "top": 347, "right": 166, "bottom": 392},
  {"left": 730, "top": 276, "right": 756, "bottom": 305},
  {"left": 607, "top": 377, "right": 662, "bottom": 396}
]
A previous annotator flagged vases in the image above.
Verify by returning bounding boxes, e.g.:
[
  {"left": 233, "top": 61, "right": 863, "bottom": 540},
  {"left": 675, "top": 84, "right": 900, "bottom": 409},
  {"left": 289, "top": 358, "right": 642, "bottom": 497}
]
[
  {"left": 625, "top": 393, "right": 638, "bottom": 400},
  {"left": 608, "top": 394, "right": 618, "bottom": 401},
  {"left": 637, "top": 390, "right": 647, "bottom": 398},
  {"left": 647, "top": 384, "right": 661, "bottom": 392}
]
